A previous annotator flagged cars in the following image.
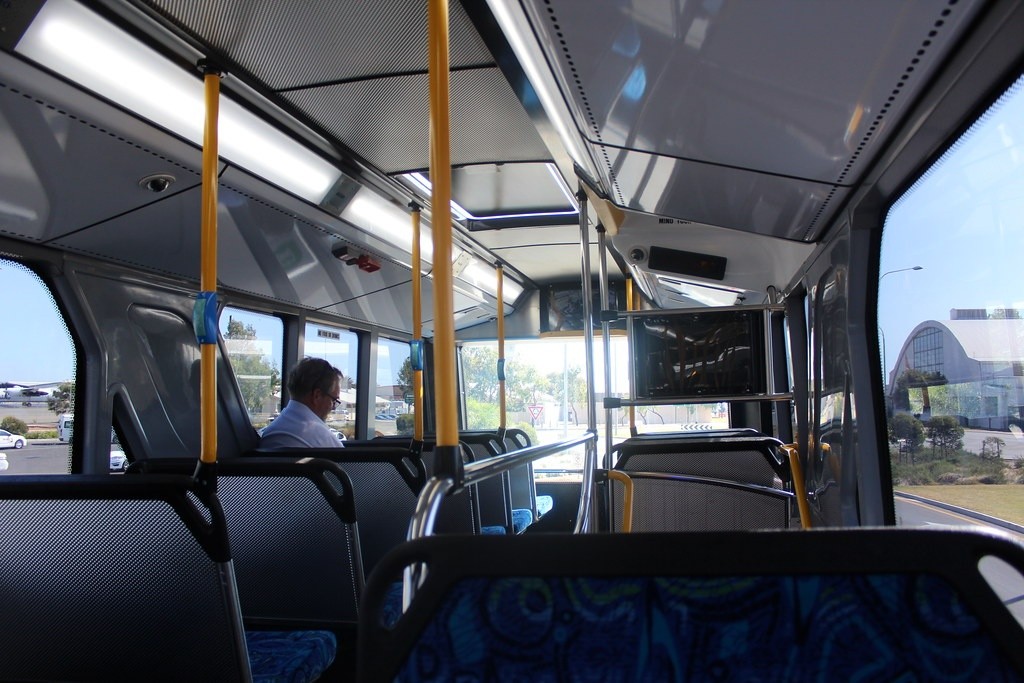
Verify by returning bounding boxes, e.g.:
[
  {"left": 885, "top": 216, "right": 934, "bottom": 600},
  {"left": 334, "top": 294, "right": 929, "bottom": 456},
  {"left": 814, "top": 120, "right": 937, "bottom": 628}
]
[
  {"left": 0, "top": 428, "right": 28, "bottom": 448},
  {"left": 110, "top": 445, "right": 130, "bottom": 472}
]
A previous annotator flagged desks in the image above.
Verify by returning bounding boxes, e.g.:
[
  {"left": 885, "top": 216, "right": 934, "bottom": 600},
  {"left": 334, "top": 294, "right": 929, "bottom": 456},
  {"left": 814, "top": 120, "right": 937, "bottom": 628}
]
[{"left": 409, "top": 428, "right": 534, "bottom": 534}]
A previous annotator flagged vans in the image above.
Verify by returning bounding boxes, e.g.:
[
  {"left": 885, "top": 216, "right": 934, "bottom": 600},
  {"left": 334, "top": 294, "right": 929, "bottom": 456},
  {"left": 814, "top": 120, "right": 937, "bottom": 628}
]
[{"left": 58, "top": 413, "right": 120, "bottom": 444}]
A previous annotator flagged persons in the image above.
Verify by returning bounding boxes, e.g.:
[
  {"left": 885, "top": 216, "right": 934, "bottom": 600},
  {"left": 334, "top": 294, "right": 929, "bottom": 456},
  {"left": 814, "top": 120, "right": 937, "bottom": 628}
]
[{"left": 258, "top": 357, "right": 344, "bottom": 448}]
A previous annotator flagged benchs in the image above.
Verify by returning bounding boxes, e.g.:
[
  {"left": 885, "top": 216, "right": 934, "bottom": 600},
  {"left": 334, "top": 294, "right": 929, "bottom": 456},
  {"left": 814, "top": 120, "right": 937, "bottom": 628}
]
[
  {"left": 601, "top": 435, "right": 797, "bottom": 530},
  {"left": 0, "top": 470, "right": 340, "bottom": 683},
  {"left": 246, "top": 444, "right": 430, "bottom": 571},
  {"left": 617, "top": 426, "right": 761, "bottom": 460},
  {"left": 128, "top": 456, "right": 403, "bottom": 626},
  {"left": 331, "top": 526, "right": 1024, "bottom": 683},
  {"left": 345, "top": 436, "right": 507, "bottom": 535},
  {"left": 461, "top": 427, "right": 555, "bottom": 522}
]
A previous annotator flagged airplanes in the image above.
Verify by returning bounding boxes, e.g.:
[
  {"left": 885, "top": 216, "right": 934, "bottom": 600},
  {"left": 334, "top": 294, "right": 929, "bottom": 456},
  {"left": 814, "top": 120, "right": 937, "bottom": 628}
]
[{"left": 0, "top": 381, "right": 62, "bottom": 407}]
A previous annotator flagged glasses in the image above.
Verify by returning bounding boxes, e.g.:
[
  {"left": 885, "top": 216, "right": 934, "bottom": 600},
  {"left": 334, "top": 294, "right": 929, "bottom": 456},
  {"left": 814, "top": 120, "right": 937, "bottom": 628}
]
[{"left": 312, "top": 386, "right": 341, "bottom": 410}]
